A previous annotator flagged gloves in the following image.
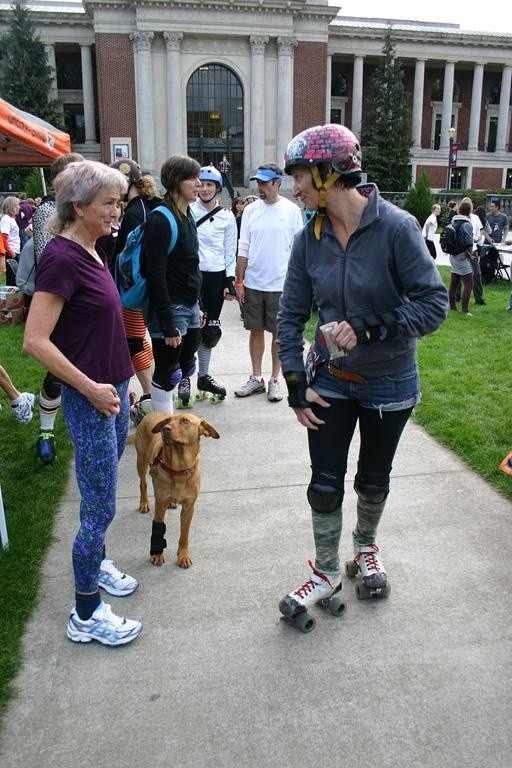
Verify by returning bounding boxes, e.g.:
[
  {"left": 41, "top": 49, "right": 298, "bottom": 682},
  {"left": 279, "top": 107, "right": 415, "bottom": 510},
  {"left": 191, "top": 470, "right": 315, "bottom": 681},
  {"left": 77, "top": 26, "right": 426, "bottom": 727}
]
[
  {"left": 284, "top": 371, "right": 315, "bottom": 408},
  {"left": 340, "top": 311, "right": 381, "bottom": 348}
]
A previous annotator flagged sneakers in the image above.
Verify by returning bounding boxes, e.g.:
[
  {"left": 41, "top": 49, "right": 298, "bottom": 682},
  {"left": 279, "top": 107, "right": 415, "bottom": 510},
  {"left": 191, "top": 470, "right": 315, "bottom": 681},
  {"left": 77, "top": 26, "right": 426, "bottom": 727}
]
[
  {"left": 262, "top": 377, "right": 285, "bottom": 405},
  {"left": 10, "top": 389, "right": 38, "bottom": 428},
  {"left": 63, "top": 597, "right": 144, "bottom": 649},
  {"left": 94, "top": 558, "right": 140, "bottom": 600},
  {"left": 231, "top": 375, "right": 265, "bottom": 400}
]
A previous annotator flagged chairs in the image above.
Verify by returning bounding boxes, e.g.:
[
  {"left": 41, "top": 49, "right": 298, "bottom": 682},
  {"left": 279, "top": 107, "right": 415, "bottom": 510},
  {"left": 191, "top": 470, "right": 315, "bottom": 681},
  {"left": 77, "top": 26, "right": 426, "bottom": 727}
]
[{"left": 476, "top": 244, "right": 510, "bottom": 286}]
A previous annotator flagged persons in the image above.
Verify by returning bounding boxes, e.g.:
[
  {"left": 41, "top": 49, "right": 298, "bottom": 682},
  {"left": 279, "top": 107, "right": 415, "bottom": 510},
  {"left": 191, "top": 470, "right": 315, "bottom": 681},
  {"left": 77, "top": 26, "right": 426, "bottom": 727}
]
[
  {"left": 233, "top": 164, "right": 303, "bottom": 400},
  {"left": 1, "top": 366, "right": 35, "bottom": 425},
  {"left": 1, "top": 198, "right": 20, "bottom": 287},
  {"left": 20, "top": 194, "right": 33, "bottom": 214},
  {"left": 465, "top": 199, "right": 487, "bottom": 305},
  {"left": 422, "top": 205, "right": 438, "bottom": 261},
  {"left": 144, "top": 154, "right": 202, "bottom": 413},
  {"left": 221, "top": 156, "right": 231, "bottom": 187},
  {"left": 33, "top": 154, "right": 85, "bottom": 461},
  {"left": 23, "top": 162, "right": 142, "bottom": 632},
  {"left": 208, "top": 161, "right": 215, "bottom": 167},
  {"left": 474, "top": 207, "right": 492, "bottom": 246},
  {"left": 449, "top": 204, "right": 475, "bottom": 314},
  {"left": 229, "top": 194, "right": 244, "bottom": 222},
  {"left": 450, "top": 201, "right": 458, "bottom": 222},
  {"left": 108, "top": 160, "right": 154, "bottom": 401},
  {"left": 175, "top": 167, "right": 239, "bottom": 406},
  {"left": 276, "top": 130, "right": 447, "bottom": 632},
  {"left": 485, "top": 201, "right": 509, "bottom": 285}
]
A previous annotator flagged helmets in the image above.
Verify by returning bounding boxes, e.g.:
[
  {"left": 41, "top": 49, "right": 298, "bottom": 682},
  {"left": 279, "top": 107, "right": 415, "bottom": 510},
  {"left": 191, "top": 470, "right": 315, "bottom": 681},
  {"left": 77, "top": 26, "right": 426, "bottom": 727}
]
[
  {"left": 107, "top": 157, "right": 145, "bottom": 189},
  {"left": 194, "top": 163, "right": 223, "bottom": 187},
  {"left": 281, "top": 123, "right": 363, "bottom": 179}
]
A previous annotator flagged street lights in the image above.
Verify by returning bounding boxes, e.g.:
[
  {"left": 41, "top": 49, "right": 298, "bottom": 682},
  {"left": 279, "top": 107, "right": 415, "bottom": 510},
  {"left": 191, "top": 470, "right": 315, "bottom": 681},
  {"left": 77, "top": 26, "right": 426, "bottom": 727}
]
[{"left": 448, "top": 127, "right": 458, "bottom": 189}]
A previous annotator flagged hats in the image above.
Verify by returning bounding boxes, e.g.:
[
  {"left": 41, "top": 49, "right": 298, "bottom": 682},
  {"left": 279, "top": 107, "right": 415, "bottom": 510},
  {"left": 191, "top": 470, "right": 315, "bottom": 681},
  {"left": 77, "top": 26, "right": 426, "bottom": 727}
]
[{"left": 250, "top": 167, "right": 281, "bottom": 187}]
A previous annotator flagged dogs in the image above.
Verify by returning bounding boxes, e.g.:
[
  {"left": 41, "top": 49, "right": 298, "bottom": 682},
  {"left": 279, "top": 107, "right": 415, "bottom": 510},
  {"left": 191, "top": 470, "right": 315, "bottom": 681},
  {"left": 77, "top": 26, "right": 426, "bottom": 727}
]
[{"left": 126, "top": 411, "right": 220, "bottom": 568}]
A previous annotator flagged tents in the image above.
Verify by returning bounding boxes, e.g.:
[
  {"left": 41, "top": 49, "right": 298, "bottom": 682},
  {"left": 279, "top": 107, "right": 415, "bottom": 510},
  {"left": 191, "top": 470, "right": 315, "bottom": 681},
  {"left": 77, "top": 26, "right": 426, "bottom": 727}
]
[{"left": 1, "top": 97, "right": 71, "bottom": 196}]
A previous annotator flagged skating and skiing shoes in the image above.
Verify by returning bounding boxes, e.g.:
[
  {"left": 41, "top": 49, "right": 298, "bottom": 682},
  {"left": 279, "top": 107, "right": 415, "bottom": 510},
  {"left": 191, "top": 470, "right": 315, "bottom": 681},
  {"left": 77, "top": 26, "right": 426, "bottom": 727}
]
[
  {"left": 274, "top": 559, "right": 347, "bottom": 633},
  {"left": 174, "top": 375, "right": 195, "bottom": 414},
  {"left": 344, "top": 545, "right": 396, "bottom": 605},
  {"left": 195, "top": 374, "right": 226, "bottom": 407},
  {"left": 35, "top": 426, "right": 61, "bottom": 467}
]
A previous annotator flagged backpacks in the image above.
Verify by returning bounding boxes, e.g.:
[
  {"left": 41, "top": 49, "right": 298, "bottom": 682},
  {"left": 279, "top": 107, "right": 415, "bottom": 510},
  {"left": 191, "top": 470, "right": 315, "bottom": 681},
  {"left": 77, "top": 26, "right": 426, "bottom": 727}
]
[
  {"left": 441, "top": 218, "right": 468, "bottom": 257},
  {"left": 115, "top": 207, "right": 177, "bottom": 310}
]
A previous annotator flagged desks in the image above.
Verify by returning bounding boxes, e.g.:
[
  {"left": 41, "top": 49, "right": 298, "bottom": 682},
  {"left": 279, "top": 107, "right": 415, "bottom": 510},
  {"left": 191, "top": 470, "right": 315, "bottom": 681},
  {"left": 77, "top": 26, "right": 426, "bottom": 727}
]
[{"left": 483, "top": 243, "right": 511, "bottom": 253}]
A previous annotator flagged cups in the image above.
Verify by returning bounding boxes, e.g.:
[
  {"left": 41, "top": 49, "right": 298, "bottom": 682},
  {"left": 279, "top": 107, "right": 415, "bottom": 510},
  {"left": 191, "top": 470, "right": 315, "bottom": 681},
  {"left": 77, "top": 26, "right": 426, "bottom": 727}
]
[{"left": 319, "top": 321, "right": 347, "bottom": 361}]
